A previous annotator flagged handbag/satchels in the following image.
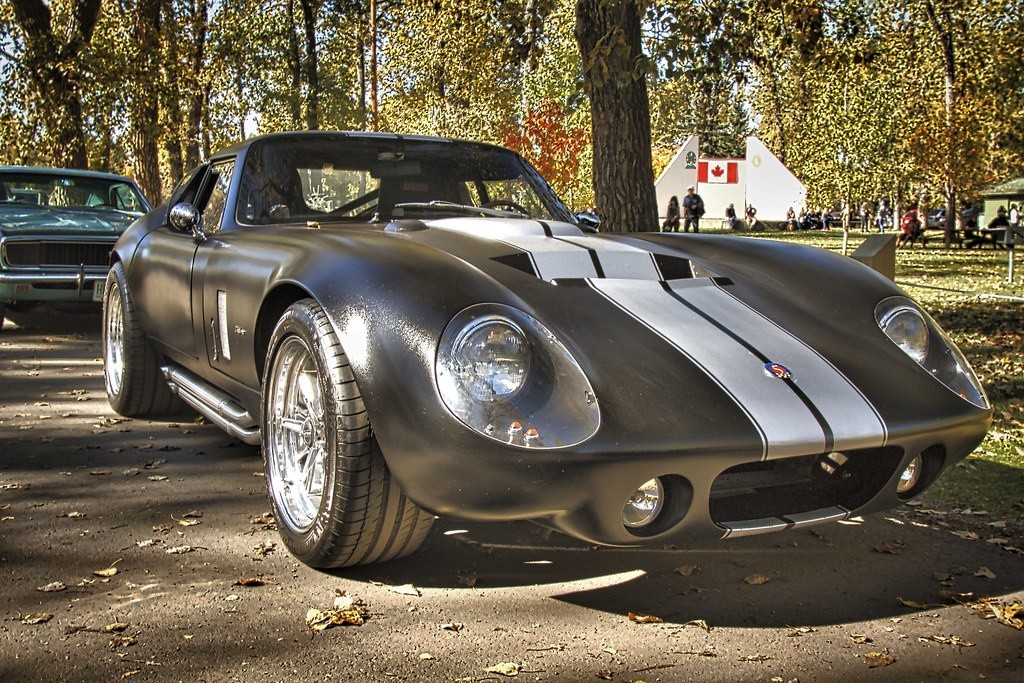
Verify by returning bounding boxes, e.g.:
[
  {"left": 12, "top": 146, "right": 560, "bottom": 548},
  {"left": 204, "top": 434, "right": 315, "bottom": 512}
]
[
  {"left": 662, "top": 219, "right": 673, "bottom": 233},
  {"left": 695, "top": 208, "right": 706, "bottom": 218}
]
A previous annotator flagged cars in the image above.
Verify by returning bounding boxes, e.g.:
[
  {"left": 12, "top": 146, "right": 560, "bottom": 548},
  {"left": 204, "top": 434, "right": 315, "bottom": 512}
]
[{"left": 0, "top": 166, "right": 156, "bottom": 329}]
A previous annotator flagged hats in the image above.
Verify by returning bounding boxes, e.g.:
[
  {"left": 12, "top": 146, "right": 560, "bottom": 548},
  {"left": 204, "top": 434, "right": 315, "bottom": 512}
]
[{"left": 687, "top": 185, "right": 695, "bottom": 191}]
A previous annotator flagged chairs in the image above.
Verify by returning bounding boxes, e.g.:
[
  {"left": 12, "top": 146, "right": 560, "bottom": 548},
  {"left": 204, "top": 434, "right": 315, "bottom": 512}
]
[
  {"left": 247, "top": 162, "right": 328, "bottom": 222},
  {"left": 896, "top": 226, "right": 1024, "bottom": 249},
  {"left": 378, "top": 162, "right": 440, "bottom": 221}
]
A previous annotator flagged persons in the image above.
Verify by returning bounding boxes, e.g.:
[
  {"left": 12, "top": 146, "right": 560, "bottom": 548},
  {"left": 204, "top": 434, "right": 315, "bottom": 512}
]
[
  {"left": 988, "top": 207, "right": 1010, "bottom": 248},
  {"left": 962, "top": 203, "right": 973, "bottom": 224},
  {"left": 876, "top": 200, "right": 886, "bottom": 233},
  {"left": 799, "top": 206, "right": 832, "bottom": 231},
  {"left": 964, "top": 215, "right": 982, "bottom": 248},
  {"left": 724, "top": 203, "right": 740, "bottom": 230},
  {"left": 662, "top": 196, "right": 679, "bottom": 232},
  {"left": 896, "top": 215, "right": 927, "bottom": 249},
  {"left": 842, "top": 204, "right": 852, "bottom": 227},
  {"left": 746, "top": 204, "right": 757, "bottom": 230},
  {"left": 682, "top": 185, "right": 703, "bottom": 233},
  {"left": 786, "top": 208, "right": 796, "bottom": 231},
  {"left": 861, "top": 202, "right": 870, "bottom": 233},
  {"left": 1010, "top": 204, "right": 1019, "bottom": 239}
]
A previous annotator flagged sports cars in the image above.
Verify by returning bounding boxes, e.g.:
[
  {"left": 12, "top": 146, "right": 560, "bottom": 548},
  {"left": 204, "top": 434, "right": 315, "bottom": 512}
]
[{"left": 102, "top": 129, "right": 994, "bottom": 567}]
